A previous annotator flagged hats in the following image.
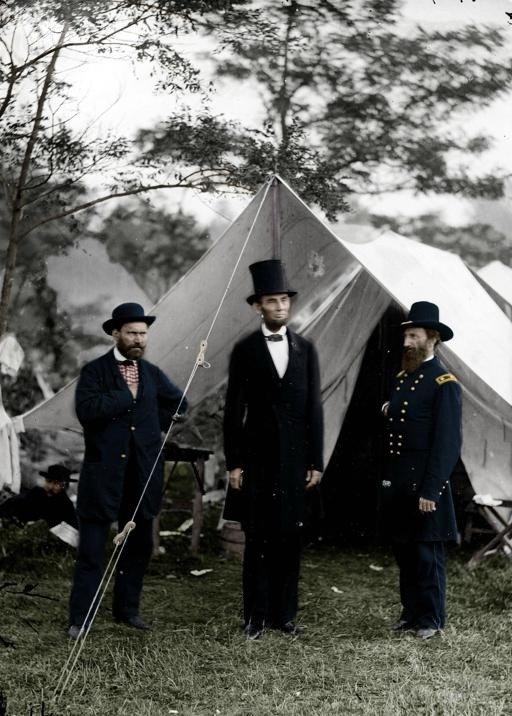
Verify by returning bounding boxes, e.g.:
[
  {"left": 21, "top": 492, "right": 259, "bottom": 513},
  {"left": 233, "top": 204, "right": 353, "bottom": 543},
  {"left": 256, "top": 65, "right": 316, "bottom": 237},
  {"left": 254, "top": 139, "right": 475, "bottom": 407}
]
[
  {"left": 39, "top": 465, "right": 78, "bottom": 483},
  {"left": 245, "top": 259, "right": 297, "bottom": 304},
  {"left": 102, "top": 303, "right": 156, "bottom": 334},
  {"left": 400, "top": 302, "right": 453, "bottom": 342}
]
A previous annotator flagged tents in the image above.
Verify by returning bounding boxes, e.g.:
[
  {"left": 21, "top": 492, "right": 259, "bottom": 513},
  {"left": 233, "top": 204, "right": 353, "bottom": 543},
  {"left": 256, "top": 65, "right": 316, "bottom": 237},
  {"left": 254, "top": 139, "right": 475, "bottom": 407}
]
[{"left": 13, "top": 166, "right": 512, "bottom": 508}]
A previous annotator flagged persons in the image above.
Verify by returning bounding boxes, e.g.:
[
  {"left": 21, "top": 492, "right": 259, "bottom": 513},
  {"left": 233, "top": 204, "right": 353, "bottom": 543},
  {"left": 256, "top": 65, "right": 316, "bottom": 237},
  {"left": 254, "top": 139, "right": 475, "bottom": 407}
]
[
  {"left": 1, "top": 464, "right": 79, "bottom": 532},
  {"left": 376, "top": 302, "right": 463, "bottom": 642},
  {"left": 65, "top": 300, "right": 191, "bottom": 636},
  {"left": 219, "top": 291, "right": 327, "bottom": 640}
]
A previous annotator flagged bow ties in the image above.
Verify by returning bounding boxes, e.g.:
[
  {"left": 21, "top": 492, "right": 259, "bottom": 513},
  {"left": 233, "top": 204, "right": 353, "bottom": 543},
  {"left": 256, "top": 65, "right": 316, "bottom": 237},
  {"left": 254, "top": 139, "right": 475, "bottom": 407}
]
[
  {"left": 269, "top": 333, "right": 282, "bottom": 340},
  {"left": 117, "top": 360, "right": 134, "bottom": 366}
]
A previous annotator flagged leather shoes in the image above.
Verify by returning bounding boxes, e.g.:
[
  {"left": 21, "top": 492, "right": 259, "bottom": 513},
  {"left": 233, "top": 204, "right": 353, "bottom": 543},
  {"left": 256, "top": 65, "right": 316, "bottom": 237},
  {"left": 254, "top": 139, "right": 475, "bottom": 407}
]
[
  {"left": 417, "top": 628, "right": 438, "bottom": 639},
  {"left": 270, "top": 622, "right": 302, "bottom": 636},
  {"left": 120, "top": 616, "right": 150, "bottom": 629},
  {"left": 389, "top": 619, "right": 418, "bottom": 630},
  {"left": 243, "top": 620, "right": 259, "bottom": 636},
  {"left": 68, "top": 624, "right": 82, "bottom": 639}
]
